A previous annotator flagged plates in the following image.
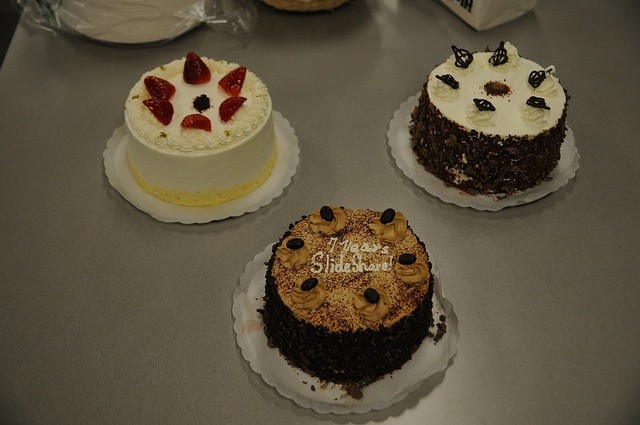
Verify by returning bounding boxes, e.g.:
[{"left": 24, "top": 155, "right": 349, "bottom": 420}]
[
  {"left": 263, "top": 0, "right": 349, "bottom": 13},
  {"left": 57, "top": 0, "right": 206, "bottom": 45}
]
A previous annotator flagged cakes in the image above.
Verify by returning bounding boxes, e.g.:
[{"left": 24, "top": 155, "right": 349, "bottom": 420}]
[
  {"left": 256, "top": 204, "right": 447, "bottom": 400},
  {"left": 123, "top": 52, "right": 279, "bottom": 207},
  {"left": 407, "top": 41, "right": 572, "bottom": 202}
]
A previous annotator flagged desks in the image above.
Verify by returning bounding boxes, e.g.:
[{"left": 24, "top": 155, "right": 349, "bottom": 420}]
[{"left": 1, "top": 0, "right": 640, "bottom": 424}]
